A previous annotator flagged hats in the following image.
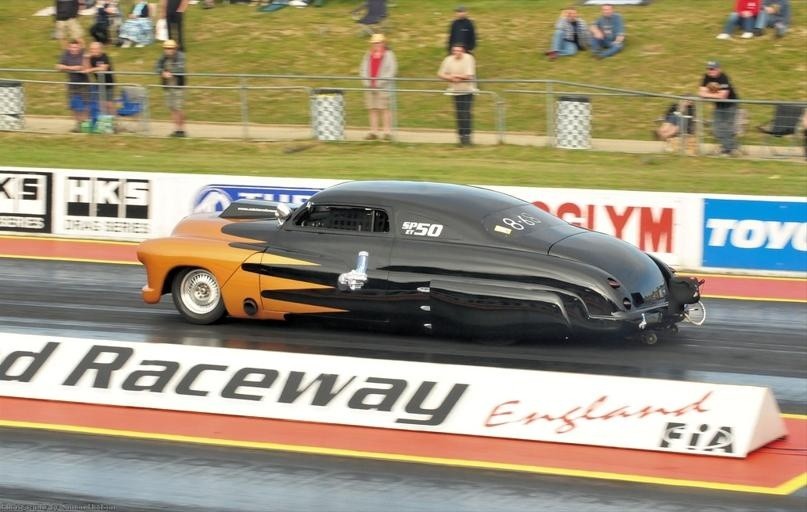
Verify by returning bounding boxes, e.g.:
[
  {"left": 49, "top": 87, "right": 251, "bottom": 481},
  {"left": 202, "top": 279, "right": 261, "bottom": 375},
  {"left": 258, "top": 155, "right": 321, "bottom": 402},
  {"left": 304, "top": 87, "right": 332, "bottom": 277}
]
[
  {"left": 163, "top": 39, "right": 176, "bottom": 47},
  {"left": 368, "top": 33, "right": 386, "bottom": 44},
  {"left": 706, "top": 61, "right": 718, "bottom": 68}
]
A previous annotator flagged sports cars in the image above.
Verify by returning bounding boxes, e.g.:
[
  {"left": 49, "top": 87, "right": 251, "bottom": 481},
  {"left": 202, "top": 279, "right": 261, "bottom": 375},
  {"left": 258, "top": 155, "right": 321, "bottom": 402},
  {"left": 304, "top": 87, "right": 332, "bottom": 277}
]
[{"left": 134, "top": 177, "right": 707, "bottom": 353}]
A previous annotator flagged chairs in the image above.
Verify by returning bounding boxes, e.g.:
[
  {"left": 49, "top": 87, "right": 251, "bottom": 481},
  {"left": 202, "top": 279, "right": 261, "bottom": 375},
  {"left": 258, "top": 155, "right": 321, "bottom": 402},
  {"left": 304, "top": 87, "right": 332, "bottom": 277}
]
[{"left": 758, "top": 103, "right": 806, "bottom": 155}]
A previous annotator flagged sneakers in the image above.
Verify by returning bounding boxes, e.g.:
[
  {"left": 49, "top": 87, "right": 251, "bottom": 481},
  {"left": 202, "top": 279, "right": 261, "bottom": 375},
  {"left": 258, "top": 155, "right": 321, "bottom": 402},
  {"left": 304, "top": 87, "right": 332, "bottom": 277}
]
[
  {"left": 545, "top": 51, "right": 556, "bottom": 59},
  {"left": 170, "top": 129, "right": 184, "bottom": 138},
  {"left": 717, "top": 28, "right": 782, "bottom": 40},
  {"left": 366, "top": 133, "right": 392, "bottom": 140}
]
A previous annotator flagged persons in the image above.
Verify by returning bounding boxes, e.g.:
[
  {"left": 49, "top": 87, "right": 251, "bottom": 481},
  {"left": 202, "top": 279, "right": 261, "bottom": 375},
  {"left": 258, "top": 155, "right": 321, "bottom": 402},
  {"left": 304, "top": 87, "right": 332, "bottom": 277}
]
[
  {"left": 53, "top": 39, "right": 97, "bottom": 134},
  {"left": 83, "top": 40, "right": 122, "bottom": 134},
  {"left": 53, "top": 0, "right": 89, "bottom": 58},
  {"left": 753, "top": 0, "right": 792, "bottom": 41},
  {"left": 717, "top": 1, "right": 761, "bottom": 38},
  {"left": 437, "top": 41, "right": 478, "bottom": 147},
  {"left": 152, "top": 40, "right": 190, "bottom": 138},
  {"left": 586, "top": 4, "right": 627, "bottom": 61},
  {"left": 653, "top": 91, "right": 701, "bottom": 143},
  {"left": 545, "top": 6, "right": 593, "bottom": 60},
  {"left": 358, "top": 32, "right": 400, "bottom": 142},
  {"left": 448, "top": 6, "right": 477, "bottom": 58},
  {"left": 80, "top": 0, "right": 220, "bottom": 55},
  {"left": 697, "top": 59, "right": 742, "bottom": 157}
]
